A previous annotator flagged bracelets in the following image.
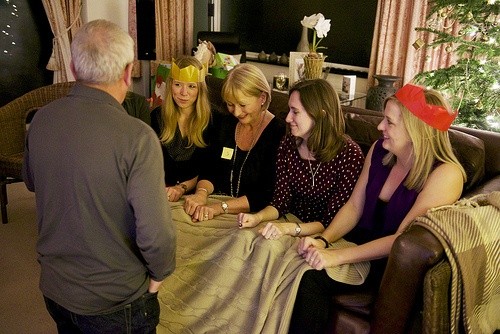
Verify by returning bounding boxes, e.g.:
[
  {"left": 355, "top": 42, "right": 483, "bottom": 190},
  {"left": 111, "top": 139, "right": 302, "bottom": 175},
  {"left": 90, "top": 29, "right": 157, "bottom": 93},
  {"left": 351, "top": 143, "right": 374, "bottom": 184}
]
[
  {"left": 314, "top": 236, "right": 329, "bottom": 248},
  {"left": 253, "top": 214, "right": 260, "bottom": 224}
]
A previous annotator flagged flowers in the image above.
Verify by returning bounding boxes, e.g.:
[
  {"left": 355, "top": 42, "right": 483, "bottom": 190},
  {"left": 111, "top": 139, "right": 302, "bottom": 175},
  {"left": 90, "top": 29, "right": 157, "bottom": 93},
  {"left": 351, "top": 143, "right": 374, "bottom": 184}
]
[{"left": 301, "top": 12, "right": 331, "bottom": 56}]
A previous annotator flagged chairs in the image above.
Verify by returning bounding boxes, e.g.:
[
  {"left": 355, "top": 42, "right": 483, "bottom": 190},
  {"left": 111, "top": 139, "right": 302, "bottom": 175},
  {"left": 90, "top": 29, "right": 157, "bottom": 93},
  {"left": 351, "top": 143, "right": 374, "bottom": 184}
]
[
  {"left": 0, "top": 82, "right": 77, "bottom": 224},
  {"left": 192, "top": 32, "right": 247, "bottom": 63}
]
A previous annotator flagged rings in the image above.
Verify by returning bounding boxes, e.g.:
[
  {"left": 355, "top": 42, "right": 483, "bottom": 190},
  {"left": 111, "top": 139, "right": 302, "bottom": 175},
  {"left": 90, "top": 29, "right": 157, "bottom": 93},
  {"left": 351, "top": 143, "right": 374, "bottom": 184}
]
[
  {"left": 203, "top": 214, "right": 209, "bottom": 217},
  {"left": 271, "top": 233, "right": 276, "bottom": 237}
]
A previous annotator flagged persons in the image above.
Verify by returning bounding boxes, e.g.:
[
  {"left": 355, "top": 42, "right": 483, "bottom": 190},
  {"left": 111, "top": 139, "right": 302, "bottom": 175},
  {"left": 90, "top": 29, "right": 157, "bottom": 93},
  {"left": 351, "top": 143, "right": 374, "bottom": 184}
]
[
  {"left": 288, "top": 84, "right": 466, "bottom": 334},
  {"left": 237, "top": 78, "right": 365, "bottom": 334},
  {"left": 183, "top": 62, "right": 287, "bottom": 223},
  {"left": 121, "top": 89, "right": 150, "bottom": 128},
  {"left": 151, "top": 55, "right": 225, "bottom": 209},
  {"left": 22, "top": 19, "right": 175, "bottom": 334}
]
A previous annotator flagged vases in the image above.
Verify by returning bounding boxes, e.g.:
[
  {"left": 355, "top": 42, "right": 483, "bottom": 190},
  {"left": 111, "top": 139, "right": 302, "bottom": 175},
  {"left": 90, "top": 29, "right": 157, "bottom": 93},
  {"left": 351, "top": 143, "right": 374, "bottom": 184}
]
[
  {"left": 366, "top": 75, "right": 400, "bottom": 112},
  {"left": 303, "top": 53, "right": 324, "bottom": 80}
]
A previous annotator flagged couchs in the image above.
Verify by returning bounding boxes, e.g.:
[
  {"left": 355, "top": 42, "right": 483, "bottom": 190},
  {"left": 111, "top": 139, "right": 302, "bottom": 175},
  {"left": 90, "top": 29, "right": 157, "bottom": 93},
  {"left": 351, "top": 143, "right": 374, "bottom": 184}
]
[{"left": 151, "top": 75, "right": 500, "bottom": 334}]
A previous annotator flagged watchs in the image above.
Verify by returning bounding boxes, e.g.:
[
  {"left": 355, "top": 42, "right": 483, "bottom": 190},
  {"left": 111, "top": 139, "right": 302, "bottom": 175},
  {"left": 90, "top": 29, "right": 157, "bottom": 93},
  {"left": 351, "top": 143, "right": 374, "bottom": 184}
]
[
  {"left": 294, "top": 222, "right": 301, "bottom": 237},
  {"left": 196, "top": 188, "right": 209, "bottom": 195},
  {"left": 179, "top": 183, "right": 188, "bottom": 192},
  {"left": 219, "top": 200, "right": 229, "bottom": 214}
]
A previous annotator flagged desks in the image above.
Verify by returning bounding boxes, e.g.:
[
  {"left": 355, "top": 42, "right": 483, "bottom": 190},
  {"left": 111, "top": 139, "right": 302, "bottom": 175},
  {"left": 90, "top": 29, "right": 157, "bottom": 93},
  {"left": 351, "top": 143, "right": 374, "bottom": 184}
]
[{"left": 269, "top": 89, "right": 367, "bottom": 107}]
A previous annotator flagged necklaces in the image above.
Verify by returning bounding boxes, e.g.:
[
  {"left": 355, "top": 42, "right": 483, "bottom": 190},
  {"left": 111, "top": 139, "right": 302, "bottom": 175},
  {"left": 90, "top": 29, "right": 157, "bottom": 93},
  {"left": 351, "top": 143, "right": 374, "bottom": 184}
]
[
  {"left": 300, "top": 138, "right": 324, "bottom": 188},
  {"left": 228, "top": 108, "right": 267, "bottom": 199}
]
